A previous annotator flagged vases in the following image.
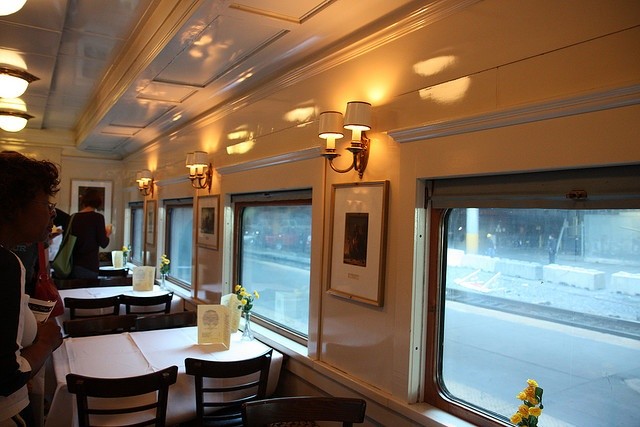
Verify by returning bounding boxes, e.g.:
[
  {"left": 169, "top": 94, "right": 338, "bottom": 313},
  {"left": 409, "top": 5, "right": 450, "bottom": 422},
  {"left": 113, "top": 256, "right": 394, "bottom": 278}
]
[
  {"left": 160, "top": 274, "right": 167, "bottom": 291},
  {"left": 123, "top": 257, "right": 127, "bottom": 267},
  {"left": 240, "top": 313, "right": 254, "bottom": 342}
]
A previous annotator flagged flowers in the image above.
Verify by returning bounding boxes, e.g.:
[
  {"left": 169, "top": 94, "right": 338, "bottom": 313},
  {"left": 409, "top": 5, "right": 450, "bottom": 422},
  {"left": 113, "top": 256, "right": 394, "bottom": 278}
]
[
  {"left": 511, "top": 380, "right": 544, "bottom": 427},
  {"left": 159, "top": 255, "right": 170, "bottom": 274},
  {"left": 122, "top": 245, "right": 129, "bottom": 256},
  {"left": 233, "top": 285, "right": 259, "bottom": 312}
]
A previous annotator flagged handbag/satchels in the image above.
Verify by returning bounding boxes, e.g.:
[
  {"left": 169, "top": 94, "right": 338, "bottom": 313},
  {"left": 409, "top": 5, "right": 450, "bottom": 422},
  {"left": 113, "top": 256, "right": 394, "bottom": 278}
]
[
  {"left": 35, "top": 268, "right": 64, "bottom": 317},
  {"left": 52, "top": 233, "right": 77, "bottom": 278}
]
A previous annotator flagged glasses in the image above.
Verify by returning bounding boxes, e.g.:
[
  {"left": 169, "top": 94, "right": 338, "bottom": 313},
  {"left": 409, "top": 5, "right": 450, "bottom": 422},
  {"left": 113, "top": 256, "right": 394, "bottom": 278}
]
[{"left": 35, "top": 201, "right": 57, "bottom": 210}]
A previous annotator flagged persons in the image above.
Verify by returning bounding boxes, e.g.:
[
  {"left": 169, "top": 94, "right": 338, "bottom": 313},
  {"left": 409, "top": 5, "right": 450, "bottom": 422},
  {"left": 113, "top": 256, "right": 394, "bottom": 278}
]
[
  {"left": 10, "top": 238, "right": 49, "bottom": 296},
  {"left": 0, "top": 150, "right": 62, "bottom": 426},
  {"left": 54, "top": 188, "right": 114, "bottom": 287}
]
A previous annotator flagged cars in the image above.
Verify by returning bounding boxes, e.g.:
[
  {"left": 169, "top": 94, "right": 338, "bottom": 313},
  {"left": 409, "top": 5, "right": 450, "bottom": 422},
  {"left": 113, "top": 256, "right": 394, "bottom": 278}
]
[{"left": 254, "top": 222, "right": 308, "bottom": 249}]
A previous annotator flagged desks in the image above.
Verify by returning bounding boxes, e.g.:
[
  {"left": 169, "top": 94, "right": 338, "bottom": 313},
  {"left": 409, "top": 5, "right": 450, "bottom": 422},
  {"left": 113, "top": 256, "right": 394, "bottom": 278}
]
[
  {"left": 53, "top": 286, "right": 185, "bottom": 338},
  {"left": 98, "top": 265, "right": 133, "bottom": 278},
  {"left": 44, "top": 325, "right": 282, "bottom": 425}
]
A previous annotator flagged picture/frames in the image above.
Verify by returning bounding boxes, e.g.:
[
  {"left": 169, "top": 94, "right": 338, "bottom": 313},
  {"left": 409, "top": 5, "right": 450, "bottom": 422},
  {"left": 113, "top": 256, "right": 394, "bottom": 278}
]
[
  {"left": 144, "top": 200, "right": 157, "bottom": 247},
  {"left": 195, "top": 194, "right": 221, "bottom": 252},
  {"left": 325, "top": 180, "right": 391, "bottom": 306}
]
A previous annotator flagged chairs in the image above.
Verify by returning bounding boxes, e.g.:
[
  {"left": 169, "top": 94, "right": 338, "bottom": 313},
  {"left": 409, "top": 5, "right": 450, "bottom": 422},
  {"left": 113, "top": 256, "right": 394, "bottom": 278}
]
[
  {"left": 65, "top": 366, "right": 178, "bottom": 427},
  {"left": 135, "top": 310, "right": 199, "bottom": 331},
  {"left": 62, "top": 312, "right": 137, "bottom": 336},
  {"left": 64, "top": 295, "right": 121, "bottom": 319},
  {"left": 238, "top": 397, "right": 367, "bottom": 427},
  {"left": 184, "top": 349, "right": 274, "bottom": 426},
  {"left": 120, "top": 291, "right": 175, "bottom": 316},
  {"left": 98, "top": 268, "right": 130, "bottom": 287}
]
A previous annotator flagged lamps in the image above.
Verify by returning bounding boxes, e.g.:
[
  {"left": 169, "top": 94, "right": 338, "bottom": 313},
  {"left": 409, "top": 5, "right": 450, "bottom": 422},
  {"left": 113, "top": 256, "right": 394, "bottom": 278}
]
[
  {"left": 184, "top": 151, "right": 209, "bottom": 189},
  {"left": 135, "top": 169, "right": 151, "bottom": 197},
  {"left": 316, "top": 101, "right": 371, "bottom": 174},
  {"left": 0, "top": 107, "right": 37, "bottom": 132},
  {"left": 0, "top": 0, "right": 29, "bottom": 16},
  {"left": 0, "top": 63, "right": 41, "bottom": 99}
]
